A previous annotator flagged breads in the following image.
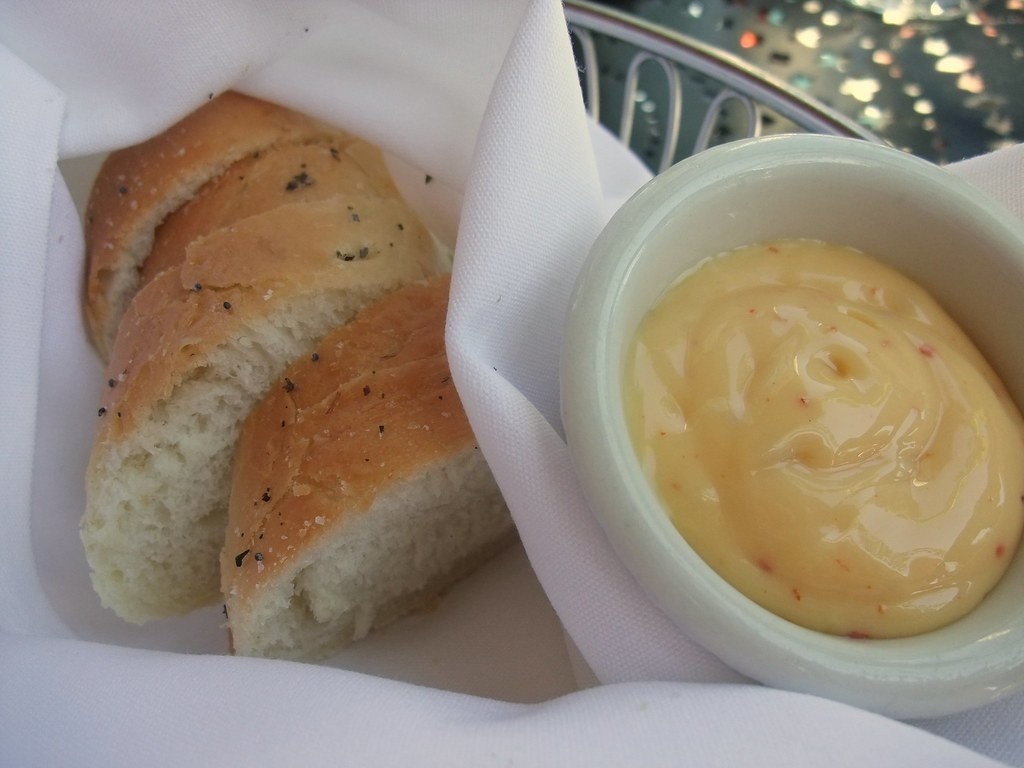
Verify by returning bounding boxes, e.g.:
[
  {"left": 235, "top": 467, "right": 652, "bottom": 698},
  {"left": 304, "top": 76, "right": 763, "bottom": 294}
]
[{"left": 79, "top": 87, "right": 517, "bottom": 660}]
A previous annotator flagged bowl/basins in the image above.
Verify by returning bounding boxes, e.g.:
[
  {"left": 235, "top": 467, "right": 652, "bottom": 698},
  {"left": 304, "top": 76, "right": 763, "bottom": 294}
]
[{"left": 557, "top": 131, "right": 1024, "bottom": 721}]
